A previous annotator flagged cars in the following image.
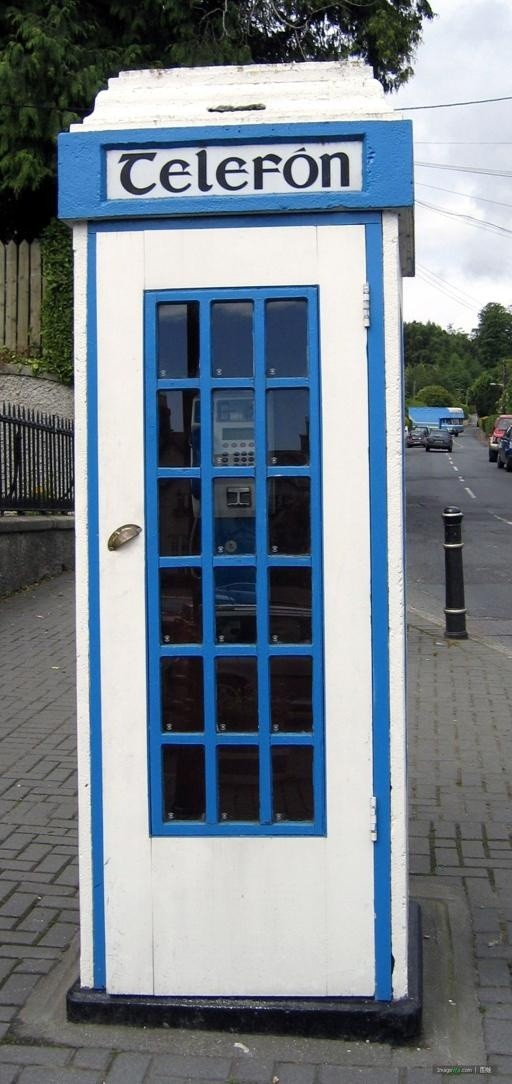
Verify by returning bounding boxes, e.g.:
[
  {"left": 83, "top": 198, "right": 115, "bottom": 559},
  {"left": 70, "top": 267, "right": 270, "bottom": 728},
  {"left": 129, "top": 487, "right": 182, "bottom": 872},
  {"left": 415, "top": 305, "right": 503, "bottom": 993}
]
[
  {"left": 155, "top": 578, "right": 314, "bottom": 771},
  {"left": 496, "top": 423, "right": 511, "bottom": 472}
]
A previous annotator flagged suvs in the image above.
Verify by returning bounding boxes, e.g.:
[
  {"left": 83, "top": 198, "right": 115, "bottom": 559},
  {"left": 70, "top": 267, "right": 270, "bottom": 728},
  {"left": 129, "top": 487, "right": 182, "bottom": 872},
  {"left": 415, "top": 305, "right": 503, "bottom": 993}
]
[
  {"left": 488, "top": 413, "right": 512, "bottom": 462},
  {"left": 407, "top": 425, "right": 454, "bottom": 453}
]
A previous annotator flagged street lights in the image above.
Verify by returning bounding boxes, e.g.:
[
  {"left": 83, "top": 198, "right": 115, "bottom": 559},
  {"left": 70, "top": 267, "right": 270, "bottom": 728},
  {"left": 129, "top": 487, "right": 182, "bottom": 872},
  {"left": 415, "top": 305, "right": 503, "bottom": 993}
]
[{"left": 489, "top": 382, "right": 506, "bottom": 414}]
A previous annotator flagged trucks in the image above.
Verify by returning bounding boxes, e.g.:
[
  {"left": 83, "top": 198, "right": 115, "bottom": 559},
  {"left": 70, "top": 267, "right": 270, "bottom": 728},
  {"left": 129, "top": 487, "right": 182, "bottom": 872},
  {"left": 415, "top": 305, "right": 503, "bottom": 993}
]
[{"left": 408, "top": 406, "right": 465, "bottom": 436}]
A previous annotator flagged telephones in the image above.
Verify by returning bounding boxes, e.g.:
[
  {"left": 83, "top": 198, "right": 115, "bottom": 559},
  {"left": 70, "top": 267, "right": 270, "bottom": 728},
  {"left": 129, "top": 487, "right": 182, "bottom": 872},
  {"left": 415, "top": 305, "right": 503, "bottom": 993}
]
[{"left": 190, "top": 390, "right": 275, "bottom": 519}]
